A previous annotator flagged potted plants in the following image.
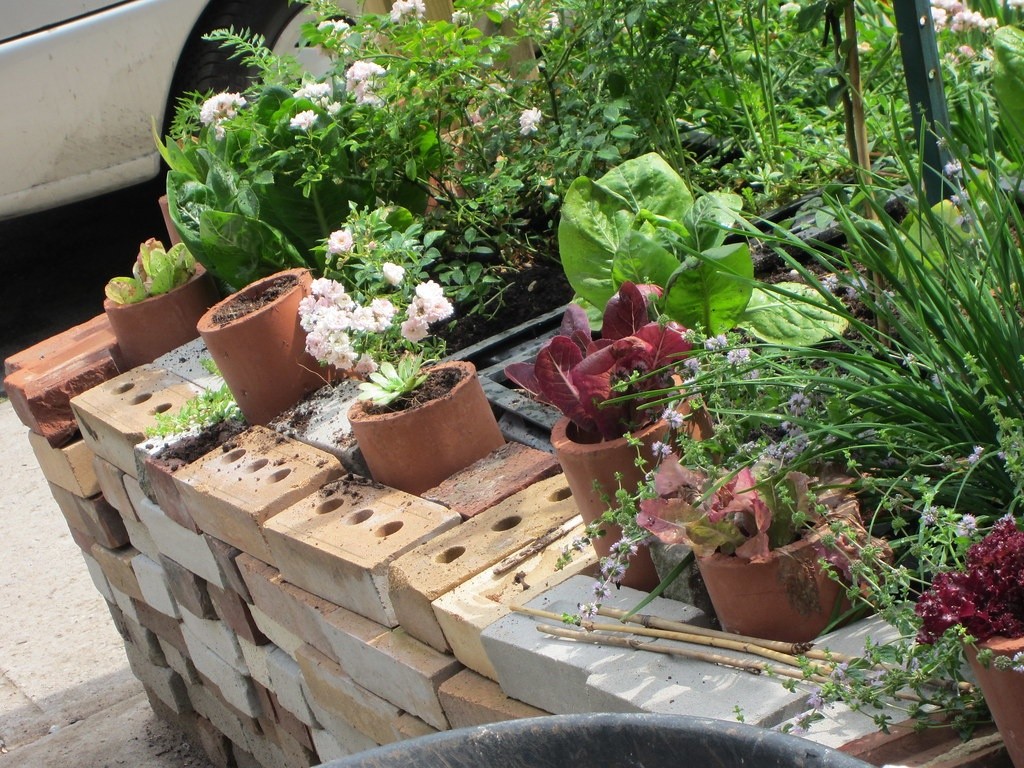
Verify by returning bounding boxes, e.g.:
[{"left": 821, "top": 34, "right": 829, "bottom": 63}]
[
  {"left": 503, "top": 278, "right": 724, "bottom": 595},
  {"left": 103, "top": 238, "right": 222, "bottom": 370},
  {"left": 636, "top": 452, "right": 880, "bottom": 647},
  {"left": 347, "top": 351, "right": 506, "bottom": 498},
  {"left": 915, "top": 515, "right": 1024, "bottom": 768}
]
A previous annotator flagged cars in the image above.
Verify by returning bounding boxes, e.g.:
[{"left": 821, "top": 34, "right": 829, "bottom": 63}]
[{"left": 0, "top": 0, "right": 558, "bottom": 291}]
